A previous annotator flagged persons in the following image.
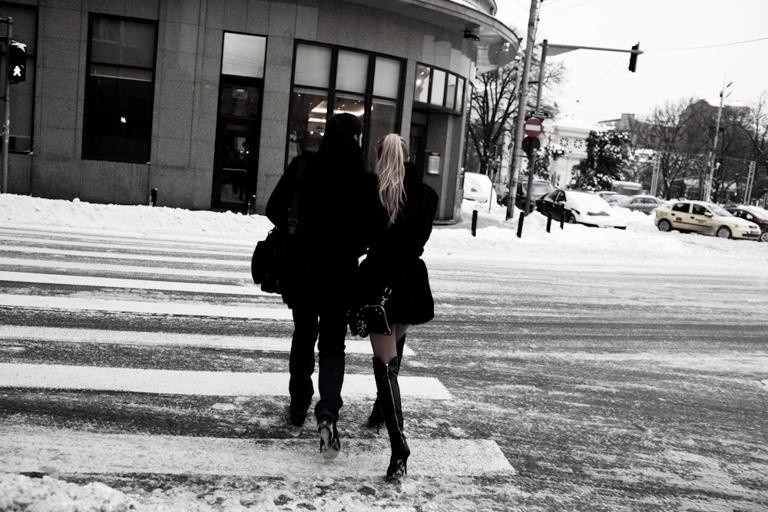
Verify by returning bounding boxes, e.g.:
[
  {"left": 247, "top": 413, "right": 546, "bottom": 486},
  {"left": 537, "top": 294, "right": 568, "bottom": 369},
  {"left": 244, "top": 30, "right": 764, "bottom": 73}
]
[
  {"left": 263, "top": 113, "right": 382, "bottom": 461},
  {"left": 342, "top": 131, "right": 440, "bottom": 484}
]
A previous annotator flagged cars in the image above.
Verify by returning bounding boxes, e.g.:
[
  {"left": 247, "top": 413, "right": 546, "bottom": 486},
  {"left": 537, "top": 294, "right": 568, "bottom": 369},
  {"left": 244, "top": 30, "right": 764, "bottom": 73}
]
[{"left": 498, "top": 179, "right": 768, "bottom": 242}]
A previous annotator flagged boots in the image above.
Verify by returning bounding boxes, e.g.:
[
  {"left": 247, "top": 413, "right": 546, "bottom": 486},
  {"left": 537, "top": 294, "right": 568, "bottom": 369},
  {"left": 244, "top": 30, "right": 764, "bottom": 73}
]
[{"left": 364, "top": 333, "right": 411, "bottom": 482}]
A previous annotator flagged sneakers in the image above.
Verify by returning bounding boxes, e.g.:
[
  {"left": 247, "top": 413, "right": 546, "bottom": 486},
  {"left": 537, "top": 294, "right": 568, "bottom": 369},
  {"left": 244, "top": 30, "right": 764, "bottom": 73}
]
[
  {"left": 316, "top": 417, "right": 342, "bottom": 461},
  {"left": 286, "top": 405, "right": 307, "bottom": 428}
]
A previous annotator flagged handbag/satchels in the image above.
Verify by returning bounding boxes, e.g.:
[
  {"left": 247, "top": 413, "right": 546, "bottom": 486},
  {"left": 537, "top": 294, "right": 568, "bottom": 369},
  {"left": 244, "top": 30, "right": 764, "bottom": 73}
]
[
  {"left": 249, "top": 224, "right": 306, "bottom": 295},
  {"left": 345, "top": 301, "right": 394, "bottom": 338}
]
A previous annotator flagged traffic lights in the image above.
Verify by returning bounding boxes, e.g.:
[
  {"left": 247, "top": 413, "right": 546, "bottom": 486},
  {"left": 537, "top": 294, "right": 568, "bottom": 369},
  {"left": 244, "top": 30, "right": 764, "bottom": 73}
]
[{"left": 7, "top": 40, "right": 27, "bottom": 84}]
